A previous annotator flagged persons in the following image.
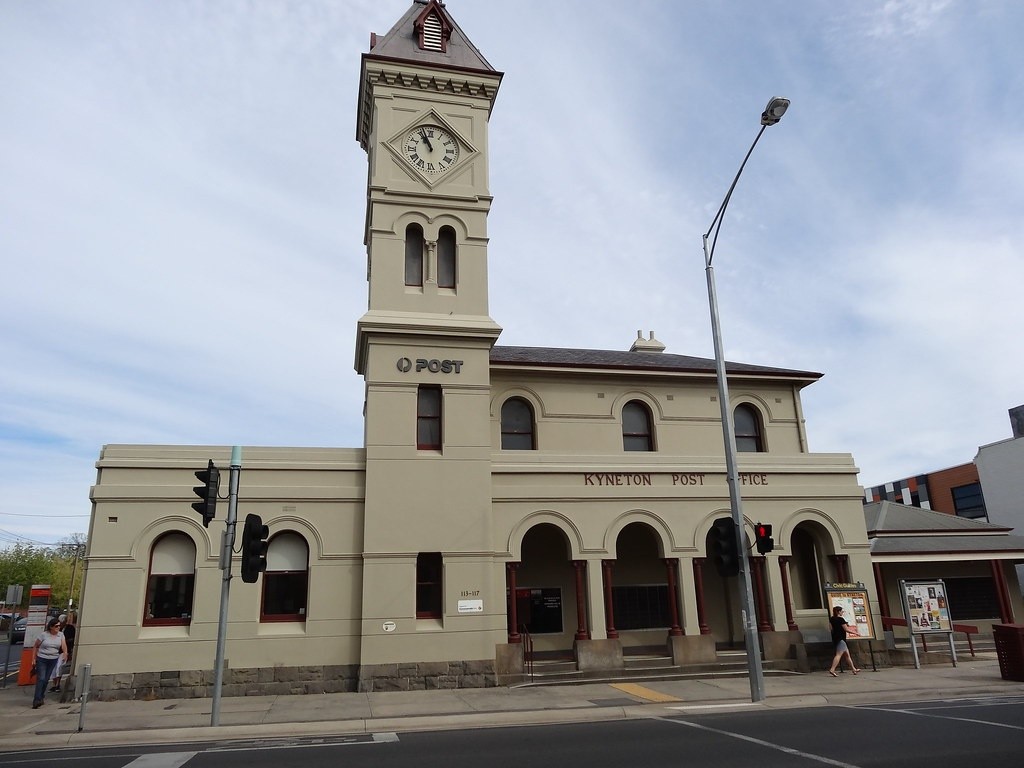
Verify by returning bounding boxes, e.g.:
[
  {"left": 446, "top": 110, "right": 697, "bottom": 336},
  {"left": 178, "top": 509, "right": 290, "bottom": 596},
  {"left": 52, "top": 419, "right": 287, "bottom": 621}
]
[
  {"left": 47, "top": 614, "right": 76, "bottom": 692},
  {"left": 31, "top": 618, "right": 68, "bottom": 710},
  {"left": 829, "top": 606, "right": 861, "bottom": 677}
]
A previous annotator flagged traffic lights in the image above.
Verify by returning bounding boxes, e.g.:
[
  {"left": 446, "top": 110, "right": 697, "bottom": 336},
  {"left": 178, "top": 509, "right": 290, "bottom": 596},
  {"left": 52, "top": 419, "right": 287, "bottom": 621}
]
[
  {"left": 191, "top": 458, "right": 220, "bottom": 528},
  {"left": 711, "top": 517, "right": 739, "bottom": 577},
  {"left": 756, "top": 523, "right": 773, "bottom": 553},
  {"left": 240, "top": 513, "right": 269, "bottom": 583}
]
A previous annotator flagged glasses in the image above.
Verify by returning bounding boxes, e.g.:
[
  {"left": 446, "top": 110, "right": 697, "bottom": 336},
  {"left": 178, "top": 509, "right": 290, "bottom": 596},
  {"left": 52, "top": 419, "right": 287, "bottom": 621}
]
[{"left": 52, "top": 625, "right": 60, "bottom": 628}]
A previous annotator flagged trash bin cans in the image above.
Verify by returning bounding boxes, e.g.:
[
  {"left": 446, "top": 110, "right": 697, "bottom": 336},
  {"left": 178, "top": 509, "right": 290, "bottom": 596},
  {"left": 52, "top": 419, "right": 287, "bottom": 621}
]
[{"left": 991, "top": 622, "right": 1024, "bottom": 682}]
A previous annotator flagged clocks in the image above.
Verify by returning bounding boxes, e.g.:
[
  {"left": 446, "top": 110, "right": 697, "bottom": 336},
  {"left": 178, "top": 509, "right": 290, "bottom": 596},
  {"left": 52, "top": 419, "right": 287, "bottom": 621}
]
[{"left": 400, "top": 122, "right": 460, "bottom": 177}]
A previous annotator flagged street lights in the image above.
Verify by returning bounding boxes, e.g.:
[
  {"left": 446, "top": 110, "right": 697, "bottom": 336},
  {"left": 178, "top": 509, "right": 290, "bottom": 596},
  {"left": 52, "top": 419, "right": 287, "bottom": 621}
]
[{"left": 702, "top": 94, "right": 793, "bottom": 706}]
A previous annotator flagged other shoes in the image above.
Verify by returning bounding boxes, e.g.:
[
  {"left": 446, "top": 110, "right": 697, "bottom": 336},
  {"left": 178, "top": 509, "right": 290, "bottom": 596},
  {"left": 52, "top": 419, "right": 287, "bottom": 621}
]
[
  {"left": 48, "top": 687, "right": 56, "bottom": 693},
  {"left": 853, "top": 669, "right": 860, "bottom": 673},
  {"left": 830, "top": 670, "right": 839, "bottom": 676},
  {"left": 55, "top": 686, "right": 61, "bottom": 692},
  {"left": 32, "top": 706, "right": 37, "bottom": 709}
]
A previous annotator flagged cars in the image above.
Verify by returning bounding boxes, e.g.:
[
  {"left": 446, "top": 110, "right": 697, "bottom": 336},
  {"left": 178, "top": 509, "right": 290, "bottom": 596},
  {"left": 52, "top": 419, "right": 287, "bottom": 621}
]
[{"left": 7, "top": 616, "right": 56, "bottom": 645}]
